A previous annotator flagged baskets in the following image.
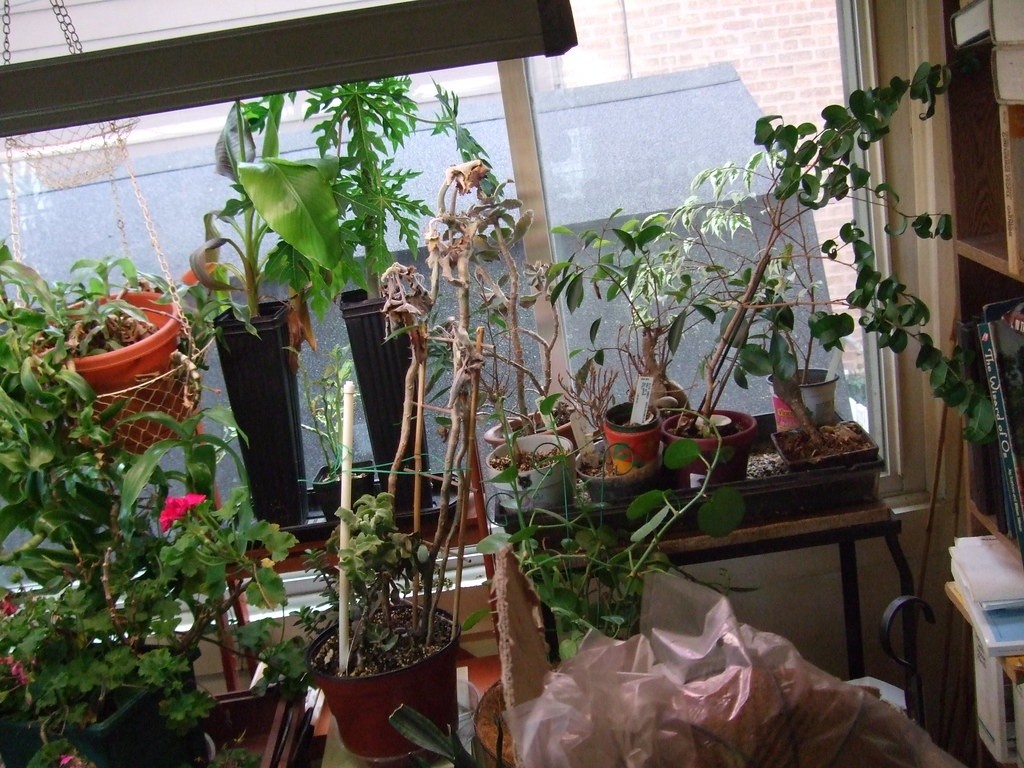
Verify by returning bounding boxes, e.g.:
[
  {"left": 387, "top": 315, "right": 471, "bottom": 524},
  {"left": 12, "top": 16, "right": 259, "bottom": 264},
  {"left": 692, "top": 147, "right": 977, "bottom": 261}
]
[
  {"left": 0, "top": 117, "right": 141, "bottom": 191},
  {"left": 57, "top": 309, "right": 217, "bottom": 454}
]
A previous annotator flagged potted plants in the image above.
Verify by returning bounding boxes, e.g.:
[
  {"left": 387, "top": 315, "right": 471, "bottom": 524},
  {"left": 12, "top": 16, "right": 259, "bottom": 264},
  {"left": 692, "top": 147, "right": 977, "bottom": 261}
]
[{"left": 0, "top": 61, "right": 997, "bottom": 768}]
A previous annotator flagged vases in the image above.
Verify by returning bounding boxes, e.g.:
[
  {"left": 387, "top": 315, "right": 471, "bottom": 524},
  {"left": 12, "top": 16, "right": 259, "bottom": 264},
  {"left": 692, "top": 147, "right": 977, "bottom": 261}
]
[{"left": 58, "top": 643, "right": 209, "bottom": 768}]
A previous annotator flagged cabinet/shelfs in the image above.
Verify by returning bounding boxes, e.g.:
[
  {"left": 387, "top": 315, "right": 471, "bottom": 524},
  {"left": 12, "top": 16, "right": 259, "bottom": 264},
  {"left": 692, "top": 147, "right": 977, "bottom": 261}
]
[{"left": 942, "top": 0, "right": 1024, "bottom": 768}]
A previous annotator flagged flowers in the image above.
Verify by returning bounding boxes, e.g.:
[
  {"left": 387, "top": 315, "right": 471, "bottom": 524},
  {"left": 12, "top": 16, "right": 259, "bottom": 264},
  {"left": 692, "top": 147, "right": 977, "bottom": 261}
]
[{"left": 0, "top": 486, "right": 309, "bottom": 768}]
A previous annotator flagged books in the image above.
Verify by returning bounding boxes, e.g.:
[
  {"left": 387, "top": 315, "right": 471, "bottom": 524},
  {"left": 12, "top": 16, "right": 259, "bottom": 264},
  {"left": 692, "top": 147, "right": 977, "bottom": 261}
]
[
  {"left": 949, "top": 0, "right": 1024, "bottom": 276},
  {"left": 947, "top": 295, "right": 1024, "bottom": 767}
]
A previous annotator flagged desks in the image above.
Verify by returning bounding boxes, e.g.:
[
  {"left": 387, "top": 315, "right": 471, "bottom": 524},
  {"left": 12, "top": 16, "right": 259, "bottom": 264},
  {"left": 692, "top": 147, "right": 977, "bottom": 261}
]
[{"left": 528, "top": 498, "right": 916, "bottom": 721}]
